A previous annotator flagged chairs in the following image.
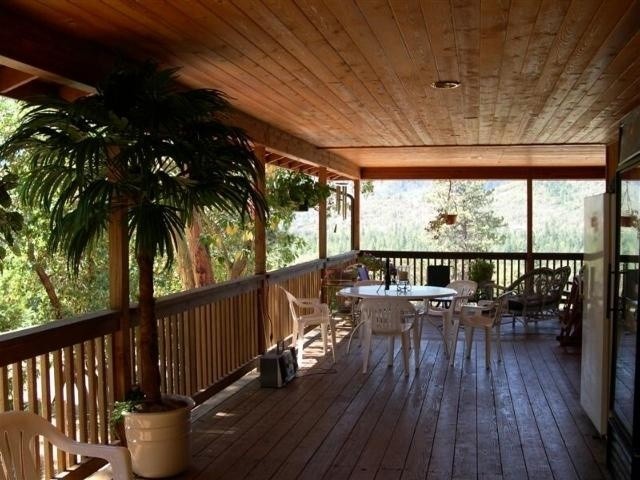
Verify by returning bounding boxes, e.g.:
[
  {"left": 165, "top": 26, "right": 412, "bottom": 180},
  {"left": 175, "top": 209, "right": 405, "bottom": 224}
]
[
  {"left": 2, "top": 408, "right": 135, "bottom": 479},
  {"left": 279, "top": 266, "right": 572, "bottom": 375}
]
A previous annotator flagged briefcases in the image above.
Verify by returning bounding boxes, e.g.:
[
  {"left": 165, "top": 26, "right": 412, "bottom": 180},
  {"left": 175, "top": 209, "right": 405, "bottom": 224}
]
[{"left": 258, "top": 340, "right": 299, "bottom": 387}]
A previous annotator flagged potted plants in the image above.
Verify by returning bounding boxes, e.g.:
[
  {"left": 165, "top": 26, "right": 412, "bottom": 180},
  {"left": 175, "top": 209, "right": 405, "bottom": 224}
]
[
  {"left": 273, "top": 168, "right": 332, "bottom": 218},
  {"left": 428, "top": 204, "right": 458, "bottom": 238},
  {"left": 1, "top": 43, "right": 272, "bottom": 480}
]
